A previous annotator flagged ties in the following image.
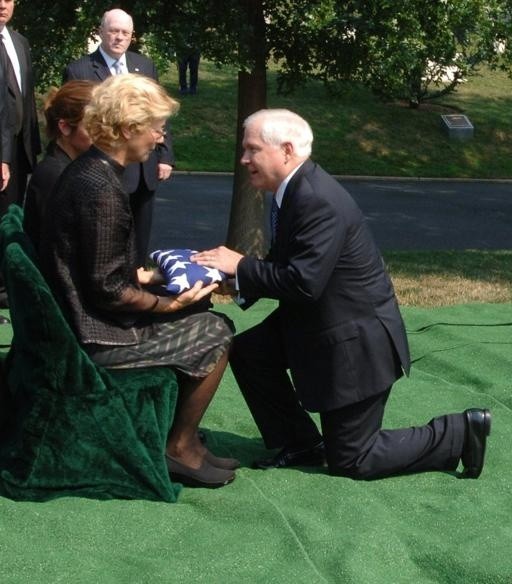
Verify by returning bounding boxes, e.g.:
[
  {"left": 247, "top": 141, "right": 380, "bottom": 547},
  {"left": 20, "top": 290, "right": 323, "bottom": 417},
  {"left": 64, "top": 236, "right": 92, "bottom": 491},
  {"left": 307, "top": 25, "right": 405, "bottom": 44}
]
[
  {"left": 269, "top": 195, "right": 281, "bottom": 243},
  {"left": 111, "top": 59, "right": 127, "bottom": 75}
]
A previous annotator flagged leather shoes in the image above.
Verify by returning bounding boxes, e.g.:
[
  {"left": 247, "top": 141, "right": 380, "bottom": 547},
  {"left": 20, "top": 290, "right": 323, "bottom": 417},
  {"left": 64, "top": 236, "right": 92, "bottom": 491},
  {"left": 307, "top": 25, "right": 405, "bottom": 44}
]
[
  {"left": 257, "top": 439, "right": 326, "bottom": 470},
  {"left": 202, "top": 447, "right": 241, "bottom": 470},
  {"left": 461, "top": 406, "right": 492, "bottom": 480},
  {"left": 163, "top": 453, "right": 237, "bottom": 489}
]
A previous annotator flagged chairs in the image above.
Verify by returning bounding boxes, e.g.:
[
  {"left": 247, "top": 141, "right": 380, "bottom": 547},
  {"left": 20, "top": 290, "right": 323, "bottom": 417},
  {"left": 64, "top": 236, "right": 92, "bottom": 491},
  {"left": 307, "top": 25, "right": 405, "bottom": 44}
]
[{"left": 0, "top": 200, "right": 185, "bottom": 504}]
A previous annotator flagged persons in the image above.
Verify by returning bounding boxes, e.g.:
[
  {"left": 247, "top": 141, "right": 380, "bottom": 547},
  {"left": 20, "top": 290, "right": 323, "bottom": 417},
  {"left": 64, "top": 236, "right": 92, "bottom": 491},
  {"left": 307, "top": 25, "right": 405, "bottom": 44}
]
[
  {"left": 36, "top": 70, "right": 242, "bottom": 491},
  {"left": 21, "top": 78, "right": 98, "bottom": 252},
  {"left": 61, "top": 7, "right": 177, "bottom": 268},
  {"left": 0, "top": 42, "right": 19, "bottom": 326},
  {"left": 188, "top": 105, "right": 493, "bottom": 482},
  {"left": 0, "top": 0, "right": 43, "bottom": 310}
]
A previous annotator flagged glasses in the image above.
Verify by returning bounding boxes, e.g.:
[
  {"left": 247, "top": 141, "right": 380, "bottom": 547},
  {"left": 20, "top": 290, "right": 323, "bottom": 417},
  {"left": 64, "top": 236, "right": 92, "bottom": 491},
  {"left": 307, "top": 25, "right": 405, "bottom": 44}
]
[{"left": 138, "top": 121, "right": 168, "bottom": 141}]
